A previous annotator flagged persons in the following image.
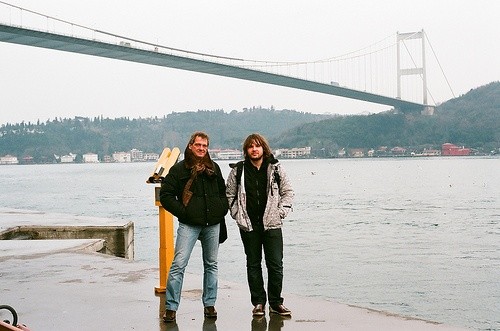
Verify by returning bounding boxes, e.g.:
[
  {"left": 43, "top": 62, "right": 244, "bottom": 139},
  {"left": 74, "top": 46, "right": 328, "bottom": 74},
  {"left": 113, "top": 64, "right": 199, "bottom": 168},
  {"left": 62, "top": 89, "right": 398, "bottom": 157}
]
[
  {"left": 160, "top": 132, "right": 228, "bottom": 321},
  {"left": 225, "top": 133, "right": 295, "bottom": 316}
]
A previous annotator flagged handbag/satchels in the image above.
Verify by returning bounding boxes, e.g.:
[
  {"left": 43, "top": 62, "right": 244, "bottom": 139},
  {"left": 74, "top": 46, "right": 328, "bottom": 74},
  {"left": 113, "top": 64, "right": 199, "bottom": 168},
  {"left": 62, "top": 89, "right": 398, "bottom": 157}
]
[{"left": 198, "top": 217, "right": 227, "bottom": 244}]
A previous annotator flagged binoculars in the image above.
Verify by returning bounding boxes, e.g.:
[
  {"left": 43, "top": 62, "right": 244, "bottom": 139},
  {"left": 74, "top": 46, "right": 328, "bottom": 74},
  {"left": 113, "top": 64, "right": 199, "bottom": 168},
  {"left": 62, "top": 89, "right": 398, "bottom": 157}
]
[{"left": 147, "top": 146, "right": 180, "bottom": 183}]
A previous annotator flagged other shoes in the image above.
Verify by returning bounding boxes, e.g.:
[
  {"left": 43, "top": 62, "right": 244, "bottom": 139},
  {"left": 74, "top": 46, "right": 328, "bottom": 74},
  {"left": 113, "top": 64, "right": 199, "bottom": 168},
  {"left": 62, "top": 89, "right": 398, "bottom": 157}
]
[
  {"left": 163, "top": 310, "right": 176, "bottom": 322},
  {"left": 252, "top": 304, "right": 266, "bottom": 316},
  {"left": 269, "top": 305, "right": 291, "bottom": 315},
  {"left": 204, "top": 306, "right": 217, "bottom": 317}
]
